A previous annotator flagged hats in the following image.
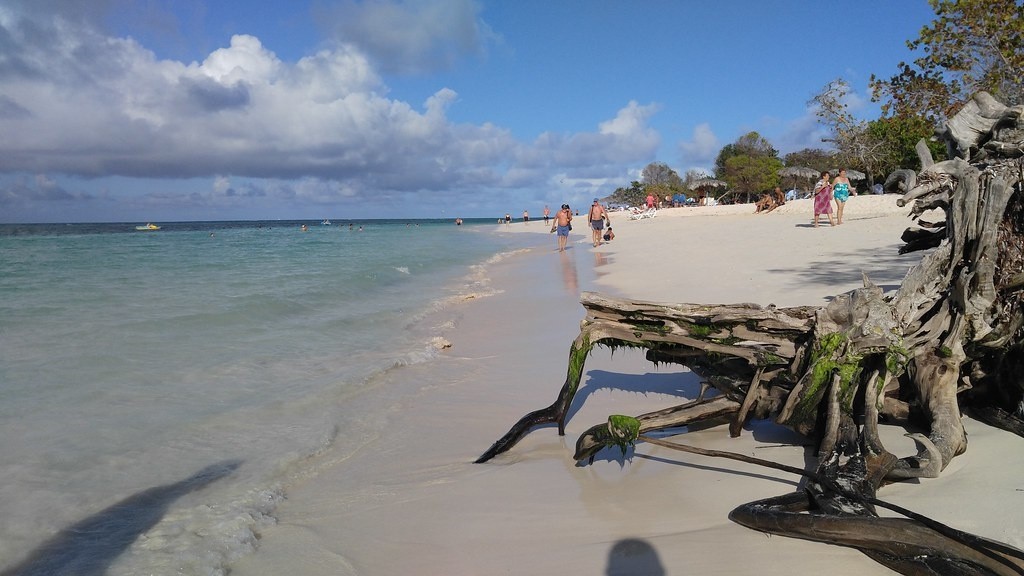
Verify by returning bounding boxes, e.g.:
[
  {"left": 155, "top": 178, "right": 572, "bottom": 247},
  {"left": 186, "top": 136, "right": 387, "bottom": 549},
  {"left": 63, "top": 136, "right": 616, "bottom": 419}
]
[
  {"left": 593, "top": 198, "right": 599, "bottom": 202},
  {"left": 562, "top": 204, "right": 568, "bottom": 209}
]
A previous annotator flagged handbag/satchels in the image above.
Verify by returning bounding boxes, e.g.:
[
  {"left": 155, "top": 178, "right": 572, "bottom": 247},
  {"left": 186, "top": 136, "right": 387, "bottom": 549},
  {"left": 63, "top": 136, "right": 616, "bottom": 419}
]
[{"left": 569, "top": 225, "right": 572, "bottom": 230}]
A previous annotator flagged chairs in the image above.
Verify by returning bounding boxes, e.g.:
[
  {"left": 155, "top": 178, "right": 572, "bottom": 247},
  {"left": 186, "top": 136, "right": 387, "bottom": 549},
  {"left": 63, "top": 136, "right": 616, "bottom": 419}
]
[
  {"left": 785, "top": 190, "right": 797, "bottom": 200},
  {"left": 627, "top": 207, "right": 658, "bottom": 221}
]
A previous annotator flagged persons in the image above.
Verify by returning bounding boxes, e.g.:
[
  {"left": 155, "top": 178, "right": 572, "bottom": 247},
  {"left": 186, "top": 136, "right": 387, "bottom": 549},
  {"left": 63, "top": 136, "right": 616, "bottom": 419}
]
[
  {"left": 576, "top": 208, "right": 579, "bottom": 216},
  {"left": 552, "top": 204, "right": 572, "bottom": 252},
  {"left": 588, "top": 197, "right": 615, "bottom": 247},
  {"left": 544, "top": 204, "right": 550, "bottom": 226},
  {"left": 524, "top": 211, "right": 528, "bottom": 222},
  {"left": 505, "top": 212, "right": 512, "bottom": 229},
  {"left": 456, "top": 217, "right": 461, "bottom": 226},
  {"left": 497, "top": 218, "right": 505, "bottom": 224},
  {"left": 301, "top": 225, "right": 307, "bottom": 231},
  {"left": 753, "top": 188, "right": 786, "bottom": 215},
  {"left": 813, "top": 169, "right": 856, "bottom": 228},
  {"left": 601, "top": 192, "right": 659, "bottom": 214}
]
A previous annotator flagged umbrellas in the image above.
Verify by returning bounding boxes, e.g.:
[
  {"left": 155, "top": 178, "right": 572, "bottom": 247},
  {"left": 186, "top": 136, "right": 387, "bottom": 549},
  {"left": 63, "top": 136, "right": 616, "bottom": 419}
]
[
  {"left": 775, "top": 166, "right": 821, "bottom": 201},
  {"left": 827, "top": 168, "right": 865, "bottom": 181},
  {"left": 687, "top": 178, "right": 726, "bottom": 207}
]
